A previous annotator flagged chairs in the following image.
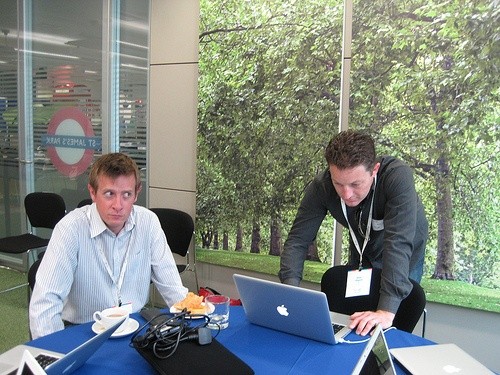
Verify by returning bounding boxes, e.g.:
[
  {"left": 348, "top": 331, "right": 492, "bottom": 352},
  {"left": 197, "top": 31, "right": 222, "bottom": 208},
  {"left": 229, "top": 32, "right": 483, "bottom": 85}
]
[
  {"left": 321, "top": 265, "right": 429, "bottom": 338},
  {"left": 0, "top": 192, "right": 199, "bottom": 340}
]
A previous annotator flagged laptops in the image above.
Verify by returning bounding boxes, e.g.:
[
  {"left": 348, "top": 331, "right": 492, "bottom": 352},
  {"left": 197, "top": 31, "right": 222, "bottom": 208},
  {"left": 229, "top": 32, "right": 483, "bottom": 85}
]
[
  {"left": 136, "top": 326, "right": 254, "bottom": 375},
  {"left": 0, "top": 314, "right": 129, "bottom": 374},
  {"left": 232, "top": 273, "right": 355, "bottom": 345}
]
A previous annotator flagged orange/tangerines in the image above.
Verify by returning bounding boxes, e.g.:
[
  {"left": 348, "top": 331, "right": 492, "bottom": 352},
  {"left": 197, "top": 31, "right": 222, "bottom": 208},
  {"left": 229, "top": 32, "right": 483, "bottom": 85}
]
[{"left": 174, "top": 291, "right": 208, "bottom": 315}]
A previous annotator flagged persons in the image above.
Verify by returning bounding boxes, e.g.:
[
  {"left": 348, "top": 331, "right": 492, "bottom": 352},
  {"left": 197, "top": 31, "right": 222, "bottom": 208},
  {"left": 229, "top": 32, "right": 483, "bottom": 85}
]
[
  {"left": 279, "top": 131, "right": 428, "bottom": 336},
  {"left": 29, "top": 153, "right": 189, "bottom": 341}
]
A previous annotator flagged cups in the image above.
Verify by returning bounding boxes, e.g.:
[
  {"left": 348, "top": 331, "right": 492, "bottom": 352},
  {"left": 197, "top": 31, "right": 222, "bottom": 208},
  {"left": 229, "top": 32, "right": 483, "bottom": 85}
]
[
  {"left": 93, "top": 308, "right": 129, "bottom": 333},
  {"left": 205, "top": 295, "right": 230, "bottom": 330}
]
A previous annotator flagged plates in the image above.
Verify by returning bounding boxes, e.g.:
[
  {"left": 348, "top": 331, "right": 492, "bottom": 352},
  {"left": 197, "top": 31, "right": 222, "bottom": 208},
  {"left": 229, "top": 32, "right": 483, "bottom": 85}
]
[
  {"left": 170, "top": 302, "right": 215, "bottom": 319},
  {"left": 91, "top": 318, "right": 139, "bottom": 338}
]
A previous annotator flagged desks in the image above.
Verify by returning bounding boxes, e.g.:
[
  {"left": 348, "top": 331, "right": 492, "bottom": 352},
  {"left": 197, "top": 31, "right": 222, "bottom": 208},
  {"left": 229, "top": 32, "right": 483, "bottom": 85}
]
[
  {"left": 0, "top": 154, "right": 60, "bottom": 234},
  {"left": 24, "top": 304, "right": 439, "bottom": 375}
]
